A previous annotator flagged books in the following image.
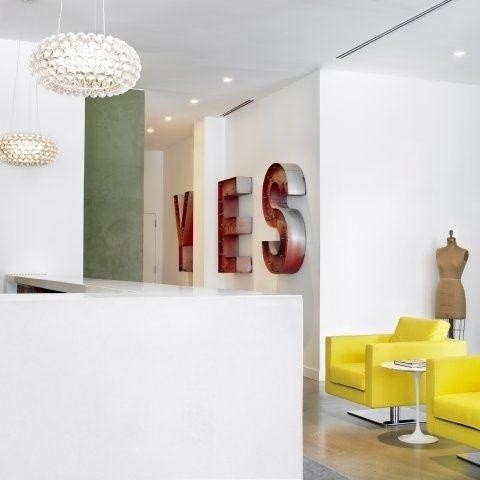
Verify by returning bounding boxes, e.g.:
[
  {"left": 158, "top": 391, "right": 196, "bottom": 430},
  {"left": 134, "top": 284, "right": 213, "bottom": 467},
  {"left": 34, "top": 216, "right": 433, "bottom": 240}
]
[{"left": 393, "top": 359, "right": 428, "bottom": 368}]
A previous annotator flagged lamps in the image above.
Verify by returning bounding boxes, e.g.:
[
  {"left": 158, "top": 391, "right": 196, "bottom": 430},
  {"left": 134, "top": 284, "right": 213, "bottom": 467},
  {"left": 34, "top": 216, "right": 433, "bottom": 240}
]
[
  {"left": 0, "top": 34, "right": 60, "bottom": 167},
  {"left": 26, "top": 0, "right": 142, "bottom": 98}
]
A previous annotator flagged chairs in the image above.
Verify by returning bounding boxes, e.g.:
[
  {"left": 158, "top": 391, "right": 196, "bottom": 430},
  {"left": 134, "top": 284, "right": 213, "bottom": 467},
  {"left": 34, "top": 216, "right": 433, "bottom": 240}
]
[
  {"left": 425, "top": 355, "right": 480, "bottom": 465},
  {"left": 324, "top": 316, "right": 467, "bottom": 425}
]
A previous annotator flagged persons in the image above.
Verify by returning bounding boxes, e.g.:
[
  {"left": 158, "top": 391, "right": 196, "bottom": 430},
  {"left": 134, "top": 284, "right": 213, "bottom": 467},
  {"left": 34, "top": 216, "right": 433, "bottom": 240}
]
[{"left": 435, "top": 234, "right": 468, "bottom": 341}]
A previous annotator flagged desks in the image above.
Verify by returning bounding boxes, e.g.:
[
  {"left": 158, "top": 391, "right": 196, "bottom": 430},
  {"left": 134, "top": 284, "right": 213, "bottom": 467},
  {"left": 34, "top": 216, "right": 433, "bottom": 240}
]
[{"left": 379, "top": 360, "right": 439, "bottom": 444}]
